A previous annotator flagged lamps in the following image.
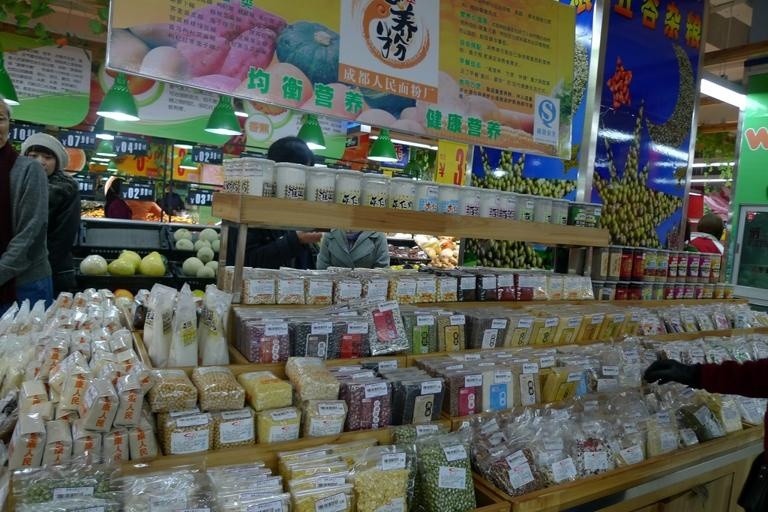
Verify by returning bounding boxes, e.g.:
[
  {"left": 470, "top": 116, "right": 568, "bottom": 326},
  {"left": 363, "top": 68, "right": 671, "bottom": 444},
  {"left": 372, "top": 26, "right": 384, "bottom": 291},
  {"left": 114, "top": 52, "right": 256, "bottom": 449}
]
[
  {"left": 701, "top": 3, "right": 747, "bottom": 110},
  {"left": 688, "top": 154, "right": 736, "bottom": 198}
]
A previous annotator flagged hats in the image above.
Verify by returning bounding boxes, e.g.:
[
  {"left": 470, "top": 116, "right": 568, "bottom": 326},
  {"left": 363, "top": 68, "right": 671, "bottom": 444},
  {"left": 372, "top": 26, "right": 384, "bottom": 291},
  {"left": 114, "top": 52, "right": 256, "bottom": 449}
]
[{"left": 21, "top": 133, "right": 68, "bottom": 170}]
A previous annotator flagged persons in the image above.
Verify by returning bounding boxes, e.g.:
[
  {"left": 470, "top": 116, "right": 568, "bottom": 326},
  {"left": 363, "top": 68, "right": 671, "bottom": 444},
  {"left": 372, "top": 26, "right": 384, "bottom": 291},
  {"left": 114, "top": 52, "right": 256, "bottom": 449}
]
[
  {"left": 643, "top": 356, "right": 768, "bottom": 511},
  {"left": 21, "top": 132, "right": 81, "bottom": 300},
  {"left": 0, "top": 99, "right": 53, "bottom": 318},
  {"left": 157, "top": 185, "right": 185, "bottom": 215},
  {"left": 225, "top": 137, "right": 322, "bottom": 269},
  {"left": 316, "top": 228, "right": 390, "bottom": 270},
  {"left": 684, "top": 213, "right": 725, "bottom": 256},
  {"left": 104, "top": 176, "right": 133, "bottom": 219}
]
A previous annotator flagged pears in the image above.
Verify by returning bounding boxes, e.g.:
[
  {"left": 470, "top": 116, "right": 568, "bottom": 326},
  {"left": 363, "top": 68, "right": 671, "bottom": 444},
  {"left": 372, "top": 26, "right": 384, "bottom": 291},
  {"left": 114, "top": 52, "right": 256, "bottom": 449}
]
[{"left": 79, "top": 248, "right": 165, "bottom": 277}]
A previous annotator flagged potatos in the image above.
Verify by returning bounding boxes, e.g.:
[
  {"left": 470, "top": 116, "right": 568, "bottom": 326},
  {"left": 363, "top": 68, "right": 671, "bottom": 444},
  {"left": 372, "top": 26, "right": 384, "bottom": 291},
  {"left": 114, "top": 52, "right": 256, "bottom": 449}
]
[{"left": 108, "top": 0, "right": 533, "bottom": 138}]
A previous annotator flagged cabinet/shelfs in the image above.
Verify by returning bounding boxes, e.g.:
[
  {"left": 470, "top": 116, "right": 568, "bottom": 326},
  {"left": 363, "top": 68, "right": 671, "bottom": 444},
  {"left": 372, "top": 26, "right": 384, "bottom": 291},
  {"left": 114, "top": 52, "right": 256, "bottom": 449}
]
[{"left": 0, "top": 150, "right": 766, "bottom": 510}]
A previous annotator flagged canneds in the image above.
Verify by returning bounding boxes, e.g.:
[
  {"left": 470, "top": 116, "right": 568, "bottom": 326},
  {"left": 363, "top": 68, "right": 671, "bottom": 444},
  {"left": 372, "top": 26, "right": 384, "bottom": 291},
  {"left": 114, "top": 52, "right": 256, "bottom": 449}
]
[
  {"left": 223, "top": 158, "right": 602, "bottom": 231},
  {"left": 591, "top": 246, "right": 734, "bottom": 301}
]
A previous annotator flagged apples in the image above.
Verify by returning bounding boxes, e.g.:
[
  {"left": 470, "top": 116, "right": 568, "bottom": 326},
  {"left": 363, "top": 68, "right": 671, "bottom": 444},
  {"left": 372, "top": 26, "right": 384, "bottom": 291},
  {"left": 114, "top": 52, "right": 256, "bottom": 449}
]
[{"left": 173, "top": 226, "right": 222, "bottom": 280}]
